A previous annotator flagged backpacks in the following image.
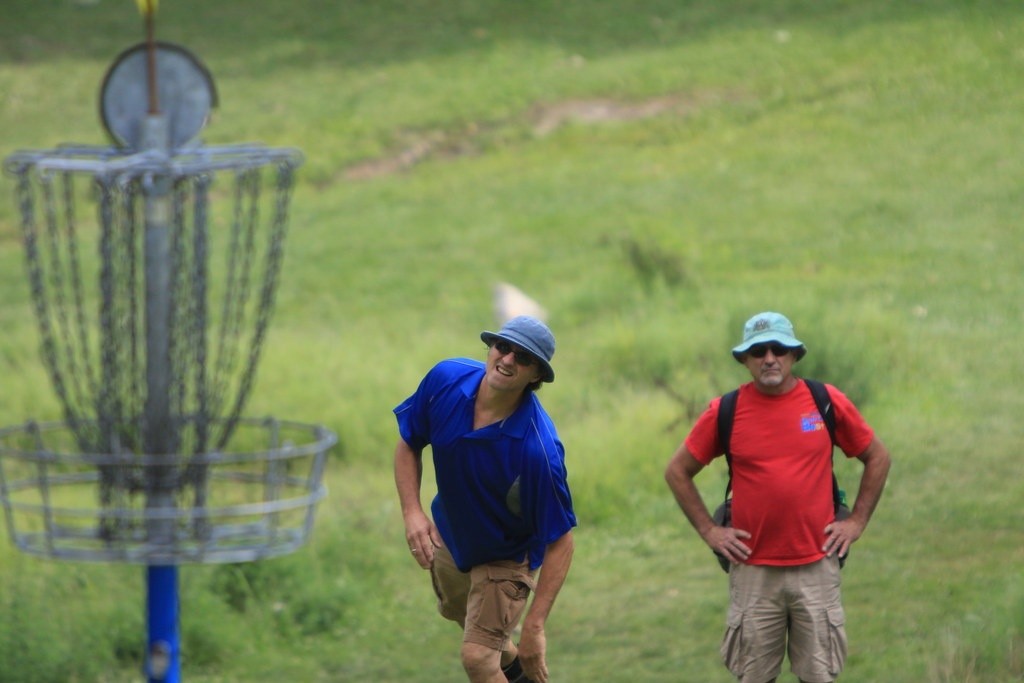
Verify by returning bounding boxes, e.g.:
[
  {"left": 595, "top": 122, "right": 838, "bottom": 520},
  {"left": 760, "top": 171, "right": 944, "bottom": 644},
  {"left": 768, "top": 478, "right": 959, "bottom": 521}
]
[{"left": 712, "top": 378, "right": 852, "bottom": 573}]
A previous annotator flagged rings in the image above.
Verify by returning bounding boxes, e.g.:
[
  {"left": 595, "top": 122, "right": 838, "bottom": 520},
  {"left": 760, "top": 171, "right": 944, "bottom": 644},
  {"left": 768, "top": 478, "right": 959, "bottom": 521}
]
[{"left": 412, "top": 549, "right": 416, "bottom": 552}]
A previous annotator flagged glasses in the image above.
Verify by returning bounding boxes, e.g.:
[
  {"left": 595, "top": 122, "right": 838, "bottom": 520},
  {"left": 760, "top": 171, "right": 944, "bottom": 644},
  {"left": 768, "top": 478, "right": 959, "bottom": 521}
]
[
  {"left": 750, "top": 345, "right": 789, "bottom": 358},
  {"left": 495, "top": 340, "right": 537, "bottom": 367}
]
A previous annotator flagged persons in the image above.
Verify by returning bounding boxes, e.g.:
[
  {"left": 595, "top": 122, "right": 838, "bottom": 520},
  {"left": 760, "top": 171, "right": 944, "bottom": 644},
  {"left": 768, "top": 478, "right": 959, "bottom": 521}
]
[
  {"left": 663, "top": 311, "right": 891, "bottom": 683},
  {"left": 392, "top": 316, "right": 578, "bottom": 683}
]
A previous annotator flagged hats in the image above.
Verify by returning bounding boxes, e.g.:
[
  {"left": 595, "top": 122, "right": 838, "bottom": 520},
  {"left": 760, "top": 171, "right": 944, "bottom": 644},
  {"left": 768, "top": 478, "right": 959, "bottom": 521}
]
[
  {"left": 731, "top": 312, "right": 807, "bottom": 364},
  {"left": 480, "top": 315, "right": 556, "bottom": 383}
]
